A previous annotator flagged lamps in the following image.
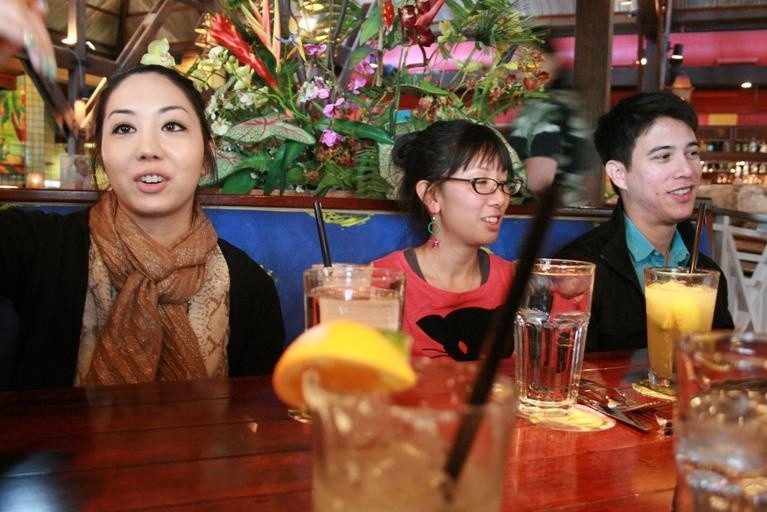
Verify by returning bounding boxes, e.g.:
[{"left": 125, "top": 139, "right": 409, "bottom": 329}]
[{"left": 666, "top": 39, "right": 683, "bottom": 59}]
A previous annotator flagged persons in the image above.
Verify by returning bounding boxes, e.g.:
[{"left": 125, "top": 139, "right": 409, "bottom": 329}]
[
  {"left": 343, "top": 119, "right": 581, "bottom": 358},
  {"left": 0, "top": 1, "right": 284, "bottom": 386},
  {"left": 526, "top": 89, "right": 734, "bottom": 354},
  {"left": 518, "top": 63, "right": 608, "bottom": 211}
]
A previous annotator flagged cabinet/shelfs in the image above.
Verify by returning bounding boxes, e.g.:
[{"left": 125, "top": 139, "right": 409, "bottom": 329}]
[{"left": 696, "top": 126, "right": 767, "bottom": 184}]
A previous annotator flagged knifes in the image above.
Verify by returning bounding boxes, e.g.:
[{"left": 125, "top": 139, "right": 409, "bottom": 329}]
[{"left": 577, "top": 394, "right": 651, "bottom": 433}]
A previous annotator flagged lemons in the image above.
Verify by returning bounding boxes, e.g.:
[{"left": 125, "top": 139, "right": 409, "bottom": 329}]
[{"left": 271, "top": 319, "right": 417, "bottom": 407}]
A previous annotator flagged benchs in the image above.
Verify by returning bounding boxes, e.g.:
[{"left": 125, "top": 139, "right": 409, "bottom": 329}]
[{"left": 0, "top": 186, "right": 716, "bottom": 389}]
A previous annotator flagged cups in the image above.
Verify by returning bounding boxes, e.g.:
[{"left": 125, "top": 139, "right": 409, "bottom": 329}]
[
  {"left": 507, "top": 256, "right": 595, "bottom": 410},
  {"left": 300, "top": 261, "right": 405, "bottom": 342},
  {"left": 671, "top": 327, "right": 766, "bottom": 511},
  {"left": 300, "top": 369, "right": 521, "bottom": 512},
  {"left": 641, "top": 265, "right": 721, "bottom": 391}
]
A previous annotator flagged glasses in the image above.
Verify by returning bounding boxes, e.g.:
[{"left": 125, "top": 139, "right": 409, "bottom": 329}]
[{"left": 426, "top": 173, "right": 523, "bottom": 198}]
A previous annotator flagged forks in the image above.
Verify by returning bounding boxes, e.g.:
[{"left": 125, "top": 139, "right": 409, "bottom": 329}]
[{"left": 578, "top": 374, "right": 675, "bottom": 425}]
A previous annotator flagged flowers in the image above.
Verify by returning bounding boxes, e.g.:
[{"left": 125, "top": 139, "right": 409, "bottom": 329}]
[{"left": 139, "top": 0, "right": 553, "bottom": 197}]
